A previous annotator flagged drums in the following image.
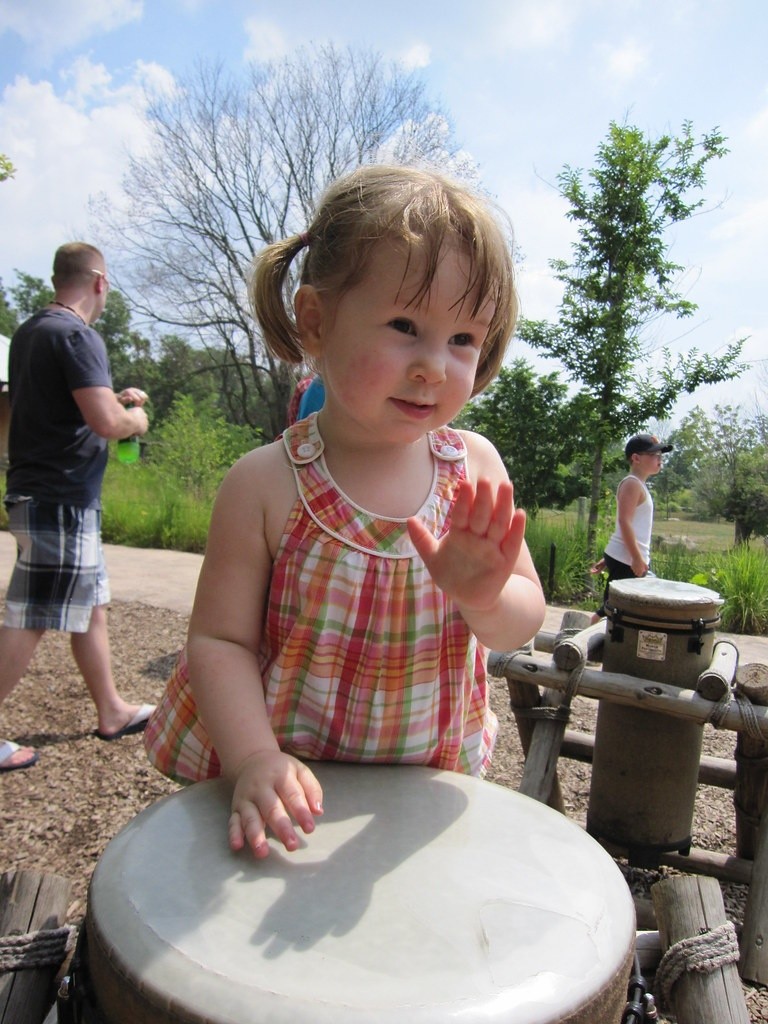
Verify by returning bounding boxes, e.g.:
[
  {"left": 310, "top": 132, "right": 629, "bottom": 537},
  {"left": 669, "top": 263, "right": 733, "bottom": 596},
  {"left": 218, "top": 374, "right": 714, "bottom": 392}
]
[
  {"left": 80, "top": 757, "right": 638, "bottom": 1022},
  {"left": 588, "top": 578, "right": 720, "bottom": 853}
]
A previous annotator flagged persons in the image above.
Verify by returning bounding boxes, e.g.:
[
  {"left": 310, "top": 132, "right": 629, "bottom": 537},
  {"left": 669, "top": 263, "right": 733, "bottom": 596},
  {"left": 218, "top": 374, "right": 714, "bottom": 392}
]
[
  {"left": 0, "top": 242, "right": 154, "bottom": 770},
  {"left": 143, "top": 164, "right": 548, "bottom": 858},
  {"left": 589, "top": 434, "right": 674, "bottom": 625}
]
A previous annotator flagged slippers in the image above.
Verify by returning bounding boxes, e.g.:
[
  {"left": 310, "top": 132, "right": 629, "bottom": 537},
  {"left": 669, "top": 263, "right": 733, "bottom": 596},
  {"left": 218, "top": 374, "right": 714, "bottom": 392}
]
[
  {"left": 92, "top": 703, "right": 157, "bottom": 740},
  {"left": 0, "top": 738, "right": 40, "bottom": 771}
]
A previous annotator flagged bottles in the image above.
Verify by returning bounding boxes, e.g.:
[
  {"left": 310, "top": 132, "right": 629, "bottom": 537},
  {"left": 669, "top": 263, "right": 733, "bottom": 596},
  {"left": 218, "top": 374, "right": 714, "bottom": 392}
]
[
  {"left": 642, "top": 570, "right": 656, "bottom": 578},
  {"left": 117, "top": 401, "right": 140, "bottom": 463}
]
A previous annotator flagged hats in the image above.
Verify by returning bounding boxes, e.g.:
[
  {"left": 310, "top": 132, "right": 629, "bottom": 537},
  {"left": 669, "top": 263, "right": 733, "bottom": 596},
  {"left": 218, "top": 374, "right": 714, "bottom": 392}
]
[{"left": 624, "top": 436, "right": 673, "bottom": 455}]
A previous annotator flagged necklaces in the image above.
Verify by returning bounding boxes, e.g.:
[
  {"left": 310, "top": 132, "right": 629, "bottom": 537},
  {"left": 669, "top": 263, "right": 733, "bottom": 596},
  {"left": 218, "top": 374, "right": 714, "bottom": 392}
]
[{"left": 48, "top": 299, "right": 89, "bottom": 328}]
